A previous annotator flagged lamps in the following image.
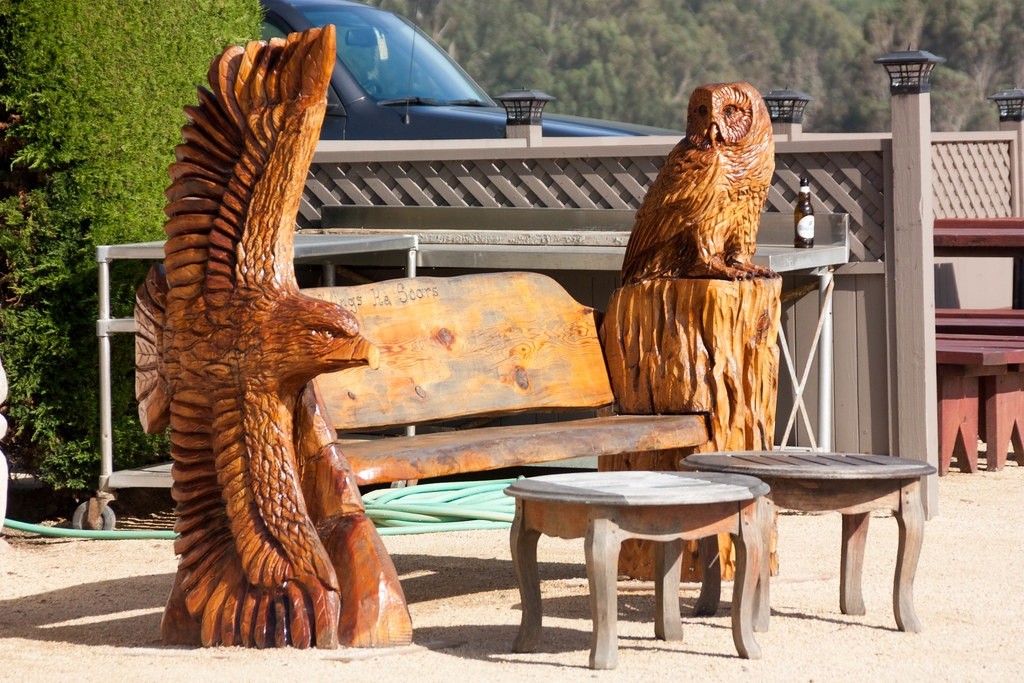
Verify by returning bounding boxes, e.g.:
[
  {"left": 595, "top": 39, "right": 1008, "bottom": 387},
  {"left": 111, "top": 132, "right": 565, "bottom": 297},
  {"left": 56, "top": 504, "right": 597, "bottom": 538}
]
[
  {"left": 874, "top": 42, "right": 947, "bottom": 95},
  {"left": 492, "top": 86, "right": 556, "bottom": 125},
  {"left": 988, "top": 89, "right": 1024, "bottom": 122},
  {"left": 762, "top": 85, "right": 815, "bottom": 124}
]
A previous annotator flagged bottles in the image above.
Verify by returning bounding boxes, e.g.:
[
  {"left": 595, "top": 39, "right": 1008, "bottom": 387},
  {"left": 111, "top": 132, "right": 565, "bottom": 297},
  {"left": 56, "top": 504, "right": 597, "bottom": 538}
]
[{"left": 794, "top": 177, "right": 815, "bottom": 248}]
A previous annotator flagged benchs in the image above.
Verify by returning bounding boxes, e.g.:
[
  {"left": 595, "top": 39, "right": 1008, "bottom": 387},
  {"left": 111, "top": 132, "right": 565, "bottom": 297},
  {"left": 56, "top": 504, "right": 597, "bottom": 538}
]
[
  {"left": 134, "top": 25, "right": 781, "bottom": 650},
  {"left": 936, "top": 307, "right": 1024, "bottom": 477}
]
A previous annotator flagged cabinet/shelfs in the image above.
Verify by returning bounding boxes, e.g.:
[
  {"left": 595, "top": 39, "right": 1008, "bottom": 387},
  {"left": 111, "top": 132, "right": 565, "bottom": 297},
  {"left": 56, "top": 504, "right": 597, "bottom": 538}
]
[{"left": 73, "top": 234, "right": 418, "bottom": 531}]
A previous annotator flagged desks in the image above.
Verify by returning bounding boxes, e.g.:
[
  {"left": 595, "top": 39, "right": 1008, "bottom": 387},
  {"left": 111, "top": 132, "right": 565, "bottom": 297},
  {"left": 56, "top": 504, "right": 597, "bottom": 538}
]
[
  {"left": 504, "top": 471, "right": 770, "bottom": 670},
  {"left": 933, "top": 217, "right": 1024, "bottom": 372},
  {"left": 296, "top": 205, "right": 850, "bottom": 455},
  {"left": 678, "top": 453, "right": 938, "bottom": 634}
]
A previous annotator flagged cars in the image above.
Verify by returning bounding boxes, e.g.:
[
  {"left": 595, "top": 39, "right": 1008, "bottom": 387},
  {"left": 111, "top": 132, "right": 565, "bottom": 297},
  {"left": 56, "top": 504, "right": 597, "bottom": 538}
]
[{"left": 260, "top": 0, "right": 687, "bottom": 142}]
